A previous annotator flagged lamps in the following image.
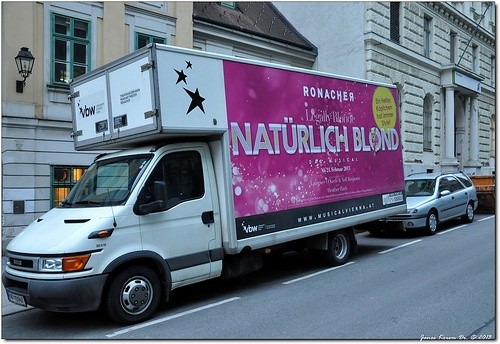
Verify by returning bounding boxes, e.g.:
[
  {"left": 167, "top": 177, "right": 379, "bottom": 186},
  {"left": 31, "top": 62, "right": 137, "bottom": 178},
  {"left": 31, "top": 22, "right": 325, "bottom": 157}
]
[{"left": 14, "top": 48, "right": 36, "bottom": 93}]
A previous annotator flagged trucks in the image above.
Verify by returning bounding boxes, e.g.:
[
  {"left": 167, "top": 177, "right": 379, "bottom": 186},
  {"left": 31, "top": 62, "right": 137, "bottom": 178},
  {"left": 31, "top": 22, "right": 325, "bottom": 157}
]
[{"left": 2, "top": 42, "right": 408, "bottom": 322}]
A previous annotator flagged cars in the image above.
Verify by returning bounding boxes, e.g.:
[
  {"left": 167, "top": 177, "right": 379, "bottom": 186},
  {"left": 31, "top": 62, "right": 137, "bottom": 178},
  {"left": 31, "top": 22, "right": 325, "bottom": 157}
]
[{"left": 370, "top": 170, "right": 478, "bottom": 234}]
